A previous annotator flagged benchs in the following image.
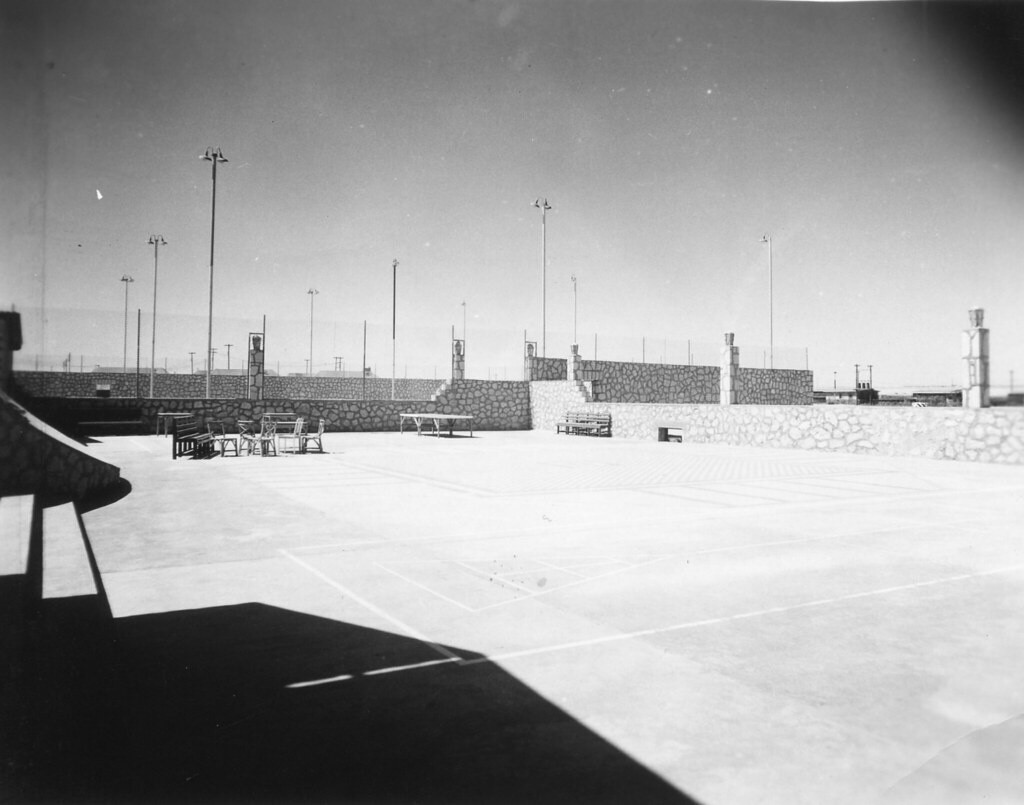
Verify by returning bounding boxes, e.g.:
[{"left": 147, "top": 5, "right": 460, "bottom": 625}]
[
  {"left": 555, "top": 411, "right": 612, "bottom": 437},
  {"left": 172, "top": 415, "right": 214, "bottom": 459}
]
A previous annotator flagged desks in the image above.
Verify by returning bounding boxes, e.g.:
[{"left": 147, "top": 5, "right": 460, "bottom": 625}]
[
  {"left": 261, "top": 422, "right": 308, "bottom": 453},
  {"left": 400, "top": 413, "right": 474, "bottom": 439},
  {"left": 156, "top": 413, "right": 192, "bottom": 438}
]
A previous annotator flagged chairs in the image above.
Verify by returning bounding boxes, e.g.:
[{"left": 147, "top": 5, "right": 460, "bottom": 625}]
[
  {"left": 278, "top": 417, "right": 304, "bottom": 454},
  {"left": 207, "top": 421, "right": 238, "bottom": 457},
  {"left": 300, "top": 419, "right": 325, "bottom": 454},
  {"left": 237, "top": 420, "right": 276, "bottom": 457}
]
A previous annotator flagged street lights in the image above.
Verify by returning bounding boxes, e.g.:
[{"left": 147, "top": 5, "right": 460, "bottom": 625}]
[
  {"left": 308, "top": 289, "right": 320, "bottom": 378},
  {"left": 758, "top": 233, "right": 774, "bottom": 369},
  {"left": 147, "top": 235, "right": 167, "bottom": 397},
  {"left": 461, "top": 298, "right": 466, "bottom": 340},
  {"left": 571, "top": 274, "right": 577, "bottom": 342},
  {"left": 531, "top": 197, "right": 551, "bottom": 358},
  {"left": 121, "top": 274, "right": 134, "bottom": 375},
  {"left": 199, "top": 145, "right": 228, "bottom": 398},
  {"left": 391, "top": 260, "right": 399, "bottom": 399}
]
